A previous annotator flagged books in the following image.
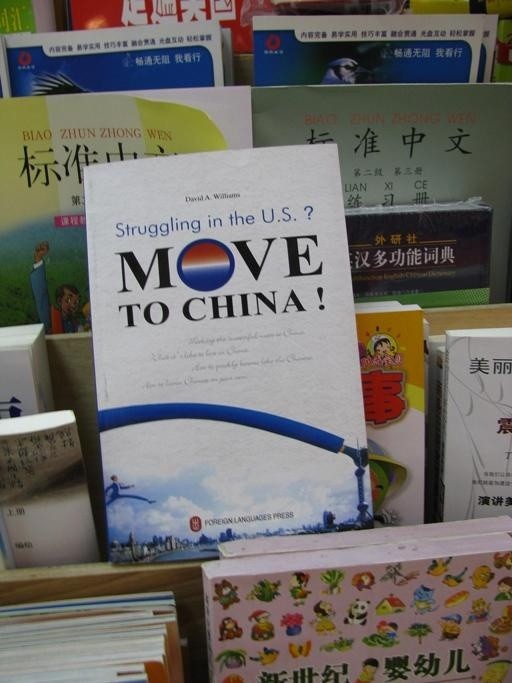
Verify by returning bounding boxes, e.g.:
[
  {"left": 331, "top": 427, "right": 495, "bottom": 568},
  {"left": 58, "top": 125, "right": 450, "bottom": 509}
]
[{"left": 1, "top": 1, "right": 511, "bottom": 683}]
